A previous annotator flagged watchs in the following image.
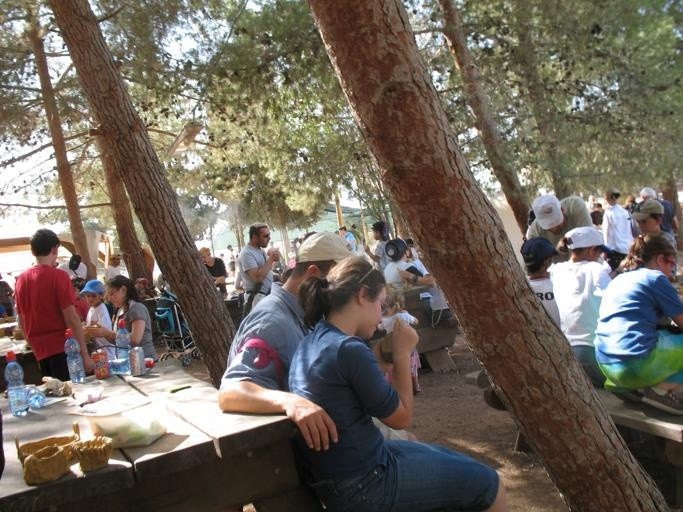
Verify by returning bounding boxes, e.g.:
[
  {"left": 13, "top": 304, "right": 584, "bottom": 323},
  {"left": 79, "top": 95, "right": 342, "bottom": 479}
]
[{"left": 411, "top": 273, "right": 418, "bottom": 285}]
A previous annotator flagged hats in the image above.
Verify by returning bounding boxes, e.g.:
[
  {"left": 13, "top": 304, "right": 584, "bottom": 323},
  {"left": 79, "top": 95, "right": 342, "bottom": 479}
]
[
  {"left": 295, "top": 230, "right": 352, "bottom": 265},
  {"left": 563, "top": 226, "right": 612, "bottom": 255},
  {"left": 109, "top": 253, "right": 123, "bottom": 260},
  {"left": 630, "top": 200, "right": 665, "bottom": 220},
  {"left": 531, "top": 195, "right": 564, "bottom": 231},
  {"left": 69, "top": 255, "right": 81, "bottom": 270},
  {"left": 80, "top": 280, "right": 105, "bottom": 295},
  {"left": 520, "top": 237, "right": 559, "bottom": 266}
]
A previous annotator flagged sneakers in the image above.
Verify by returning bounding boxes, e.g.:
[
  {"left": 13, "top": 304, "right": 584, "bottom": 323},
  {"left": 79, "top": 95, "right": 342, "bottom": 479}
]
[
  {"left": 614, "top": 385, "right": 642, "bottom": 402},
  {"left": 640, "top": 385, "right": 683, "bottom": 415}
]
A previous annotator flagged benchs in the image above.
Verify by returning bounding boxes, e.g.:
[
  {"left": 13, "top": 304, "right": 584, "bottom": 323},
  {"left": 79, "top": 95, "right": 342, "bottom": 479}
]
[{"left": 515, "top": 376, "right": 683, "bottom": 451}]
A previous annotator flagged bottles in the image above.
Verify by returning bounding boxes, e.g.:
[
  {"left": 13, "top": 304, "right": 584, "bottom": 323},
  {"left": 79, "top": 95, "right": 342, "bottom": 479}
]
[
  {"left": 92, "top": 351, "right": 110, "bottom": 379},
  {"left": 5, "top": 351, "right": 29, "bottom": 416},
  {"left": 64, "top": 329, "right": 86, "bottom": 383},
  {"left": 108, "top": 359, "right": 131, "bottom": 374},
  {"left": 128, "top": 347, "right": 146, "bottom": 376},
  {"left": 116, "top": 319, "right": 131, "bottom": 359},
  {"left": 26, "top": 386, "right": 46, "bottom": 408}
]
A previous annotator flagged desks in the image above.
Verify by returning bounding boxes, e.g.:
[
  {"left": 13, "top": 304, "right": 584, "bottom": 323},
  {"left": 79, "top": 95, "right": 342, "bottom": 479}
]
[
  {"left": 1, "top": 330, "right": 52, "bottom": 386},
  {"left": 1, "top": 360, "right": 308, "bottom": 511}
]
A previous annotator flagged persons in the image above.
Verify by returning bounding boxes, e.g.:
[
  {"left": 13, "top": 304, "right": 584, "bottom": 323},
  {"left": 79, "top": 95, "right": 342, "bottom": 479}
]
[
  {"left": 630, "top": 199, "right": 677, "bottom": 287},
  {"left": 79, "top": 279, "right": 112, "bottom": 348},
  {"left": 287, "top": 256, "right": 506, "bottom": 512},
  {"left": 520, "top": 238, "right": 561, "bottom": 334},
  {"left": 338, "top": 227, "right": 358, "bottom": 256},
  {"left": 83, "top": 275, "right": 156, "bottom": 363},
  {"left": 362, "top": 222, "right": 391, "bottom": 274},
  {"left": 550, "top": 227, "right": 613, "bottom": 390},
  {"left": 199, "top": 247, "right": 228, "bottom": 299},
  {"left": 134, "top": 278, "right": 157, "bottom": 300},
  {"left": 640, "top": 187, "right": 679, "bottom": 237},
  {"left": 351, "top": 224, "right": 363, "bottom": 246},
  {"left": 157, "top": 274, "right": 167, "bottom": 293},
  {"left": 525, "top": 194, "right": 592, "bottom": 247},
  {"left": 105, "top": 254, "right": 126, "bottom": 283},
  {"left": 593, "top": 231, "right": 683, "bottom": 417},
  {"left": 375, "top": 284, "right": 421, "bottom": 396},
  {"left": 622, "top": 196, "right": 637, "bottom": 215},
  {"left": 590, "top": 203, "right": 605, "bottom": 226},
  {"left": 602, "top": 187, "right": 633, "bottom": 271},
  {"left": 384, "top": 237, "right": 456, "bottom": 320},
  {"left": 57, "top": 254, "right": 88, "bottom": 281},
  {"left": 69, "top": 278, "right": 90, "bottom": 323},
  {"left": 225, "top": 245, "right": 237, "bottom": 278},
  {"left": 237, "top": 224, "right": 281, "bottom": 320},
  {"left": 0, "top": 273, "right": 16, "bottom": 316},
  {"left": 15, "top": 230, "right": 96, "bottom": 382},
  {"left": 218, "top": 232, "right": 355, "bottom": 452}
]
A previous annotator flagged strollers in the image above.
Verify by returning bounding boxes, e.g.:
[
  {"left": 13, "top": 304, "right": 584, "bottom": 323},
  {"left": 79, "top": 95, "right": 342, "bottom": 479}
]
[{"left": 156, "top": 297, "right": 201, "bottom": 366}]
